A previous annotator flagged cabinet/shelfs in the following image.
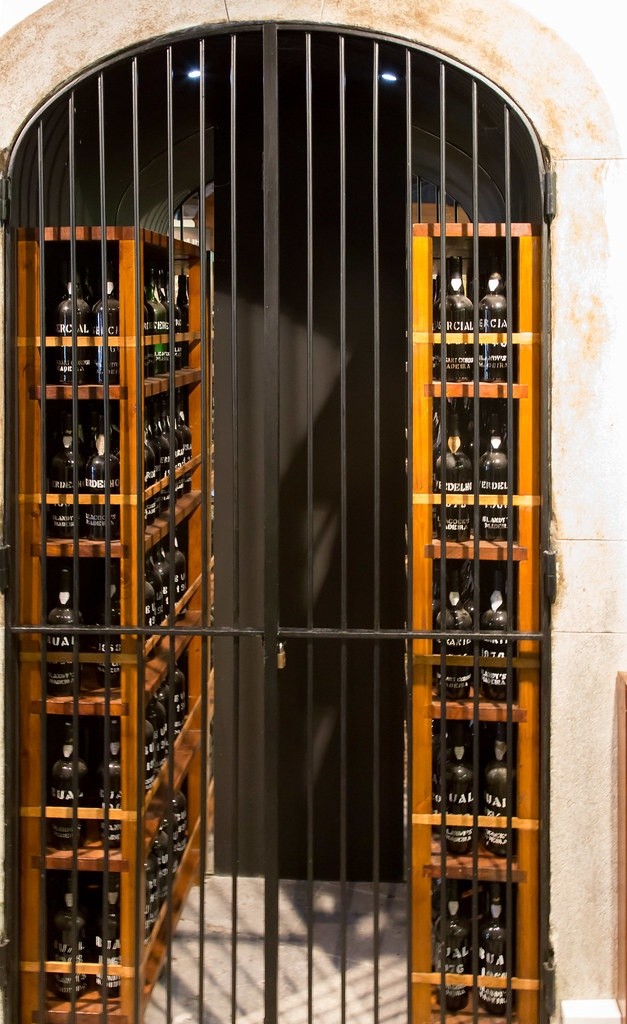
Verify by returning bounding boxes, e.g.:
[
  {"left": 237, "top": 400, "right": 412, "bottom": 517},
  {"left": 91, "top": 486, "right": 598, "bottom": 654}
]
[
  {"left": 17, "top": 225, "right": 214, "bottom": 1024},
  {"left": 408, "top": 221, "right": 548, "bottom": 1024}
]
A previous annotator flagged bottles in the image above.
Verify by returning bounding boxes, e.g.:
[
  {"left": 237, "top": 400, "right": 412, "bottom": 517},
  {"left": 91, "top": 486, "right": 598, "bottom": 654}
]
[
  {"left": 430, "top": 256, "right": 515, "bottom": 1017},
  {"left": 51, "top": 268, "right": 190, "bottom": 1002}
]
